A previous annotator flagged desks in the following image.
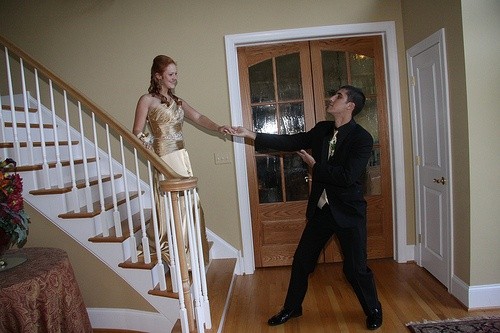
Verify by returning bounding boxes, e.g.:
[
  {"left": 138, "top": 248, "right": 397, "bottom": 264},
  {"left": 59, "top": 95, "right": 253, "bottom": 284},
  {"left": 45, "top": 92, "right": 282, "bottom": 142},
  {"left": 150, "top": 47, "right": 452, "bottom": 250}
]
[{"left": 0, "top": 247, "right": 93, "bottom": 333}]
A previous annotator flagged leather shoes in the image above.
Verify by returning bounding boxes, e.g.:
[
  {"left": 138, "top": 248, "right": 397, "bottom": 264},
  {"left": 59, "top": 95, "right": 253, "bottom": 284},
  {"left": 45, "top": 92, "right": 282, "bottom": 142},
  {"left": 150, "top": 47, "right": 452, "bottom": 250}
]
[
  {"left": 267, "top": 305, "right": 303, "bottom": 326},
  {"left": 366, "top": 304, "right": 383, "bottom": 330}
]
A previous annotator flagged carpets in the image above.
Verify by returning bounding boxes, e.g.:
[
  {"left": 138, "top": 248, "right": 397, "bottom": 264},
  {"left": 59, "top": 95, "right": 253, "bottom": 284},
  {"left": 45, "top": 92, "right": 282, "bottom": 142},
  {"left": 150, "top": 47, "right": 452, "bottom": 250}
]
[{"left": 405, "top": 315, "right": 500, "bottom": 333}]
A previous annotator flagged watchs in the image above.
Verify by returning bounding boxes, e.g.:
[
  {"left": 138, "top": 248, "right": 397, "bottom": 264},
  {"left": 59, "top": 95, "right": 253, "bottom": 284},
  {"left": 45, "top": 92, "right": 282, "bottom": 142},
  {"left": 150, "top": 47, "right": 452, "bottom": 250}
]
[{"left": 139, "top": 134, "right": 145, "bottom": 140}]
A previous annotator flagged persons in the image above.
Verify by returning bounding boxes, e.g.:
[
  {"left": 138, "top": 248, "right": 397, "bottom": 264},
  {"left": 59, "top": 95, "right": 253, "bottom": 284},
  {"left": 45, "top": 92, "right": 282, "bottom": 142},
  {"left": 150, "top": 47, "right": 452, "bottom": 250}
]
[
  {"left": 235, "top": 87, "right": 384, "bottom": 330},
  {"left": 133, "top": 55, "right": 237, "bottom": 273}
]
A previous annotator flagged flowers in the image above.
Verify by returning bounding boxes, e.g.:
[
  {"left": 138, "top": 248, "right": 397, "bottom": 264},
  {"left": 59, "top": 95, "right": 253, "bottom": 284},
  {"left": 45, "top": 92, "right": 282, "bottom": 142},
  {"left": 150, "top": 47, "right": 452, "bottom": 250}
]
[{"left": 0, "top": 157, "right": 31, "bottom": 270}]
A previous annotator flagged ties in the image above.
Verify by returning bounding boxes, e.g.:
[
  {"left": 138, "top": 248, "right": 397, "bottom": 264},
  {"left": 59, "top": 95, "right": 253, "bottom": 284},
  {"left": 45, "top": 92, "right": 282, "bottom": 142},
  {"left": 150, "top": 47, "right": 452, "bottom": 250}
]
[{"left": 326, "top": 129, "right": 338, "bottom": 160}]
